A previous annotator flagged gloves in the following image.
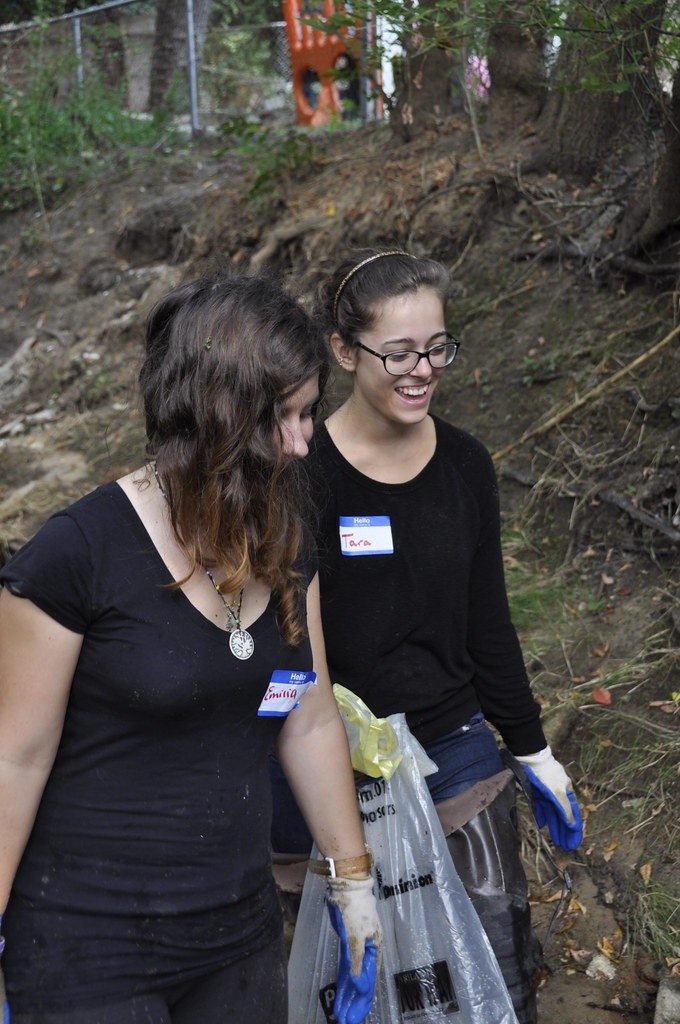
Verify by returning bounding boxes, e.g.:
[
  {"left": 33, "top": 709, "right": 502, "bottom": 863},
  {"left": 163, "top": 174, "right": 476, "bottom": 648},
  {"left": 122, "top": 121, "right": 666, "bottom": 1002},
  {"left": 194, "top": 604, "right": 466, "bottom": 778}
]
[
  {"left": 509, "top": 745, "right": 582, "bottom": 854},
  {"left": 0, "top": 936, "right": 10, "bottom": 1024},
  {"left": 324, "top": 873, "right": 384, "bottom": 1023}
]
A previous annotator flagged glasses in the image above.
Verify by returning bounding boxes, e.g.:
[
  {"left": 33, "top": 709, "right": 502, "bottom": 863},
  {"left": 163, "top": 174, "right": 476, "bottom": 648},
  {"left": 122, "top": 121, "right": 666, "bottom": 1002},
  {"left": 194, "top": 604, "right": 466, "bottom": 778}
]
[{"left": 352, "top": 331, "right": 461, "bottom": 377}]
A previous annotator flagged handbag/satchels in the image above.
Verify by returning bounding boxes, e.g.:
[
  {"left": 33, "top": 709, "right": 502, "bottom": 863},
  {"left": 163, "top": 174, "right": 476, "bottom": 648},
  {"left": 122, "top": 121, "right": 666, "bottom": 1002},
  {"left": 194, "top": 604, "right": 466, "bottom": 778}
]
[{"left": 280, "top": 681, "right": 521, "bottom": 1024}]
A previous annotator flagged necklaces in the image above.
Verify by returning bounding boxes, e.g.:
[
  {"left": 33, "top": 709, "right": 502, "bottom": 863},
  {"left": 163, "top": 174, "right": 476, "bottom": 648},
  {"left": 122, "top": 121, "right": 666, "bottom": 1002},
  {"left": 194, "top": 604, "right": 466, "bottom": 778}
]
[{"left": 155, "top": 459, "right": 261, "bottom": 664}]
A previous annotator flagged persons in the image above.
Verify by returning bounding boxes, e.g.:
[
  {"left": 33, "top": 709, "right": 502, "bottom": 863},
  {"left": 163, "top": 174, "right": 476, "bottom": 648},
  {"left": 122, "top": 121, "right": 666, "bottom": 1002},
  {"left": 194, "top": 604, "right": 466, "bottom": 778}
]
[
  {"left": 0, "top": 274, "right": 395, "bottom": 1022},
  {"left": 271, "top": 247, "right": 590, "bottom": 1024}
]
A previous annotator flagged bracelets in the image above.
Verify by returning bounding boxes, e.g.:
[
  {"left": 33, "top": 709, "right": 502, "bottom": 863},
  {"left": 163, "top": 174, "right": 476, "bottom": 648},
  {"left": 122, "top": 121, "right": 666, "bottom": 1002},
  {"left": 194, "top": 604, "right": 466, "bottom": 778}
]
[{"left": 307, "top": 851, "right": 375, "bottom": 879}]
